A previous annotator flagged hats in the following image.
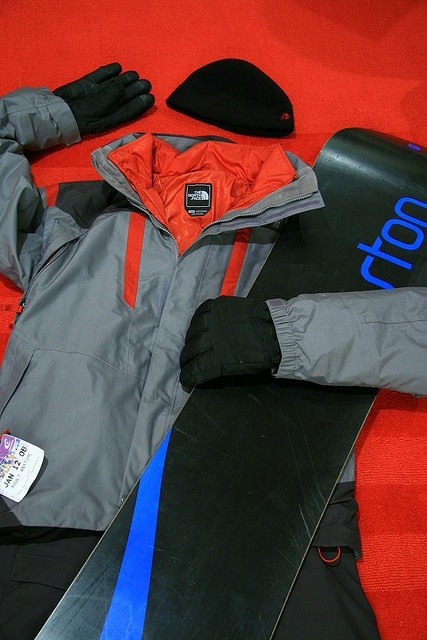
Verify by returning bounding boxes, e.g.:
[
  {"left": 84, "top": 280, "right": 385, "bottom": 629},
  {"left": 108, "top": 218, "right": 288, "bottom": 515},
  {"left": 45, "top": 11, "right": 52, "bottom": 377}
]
[{"left": 166, "top": 57, "right": 294, "bottom": 138}]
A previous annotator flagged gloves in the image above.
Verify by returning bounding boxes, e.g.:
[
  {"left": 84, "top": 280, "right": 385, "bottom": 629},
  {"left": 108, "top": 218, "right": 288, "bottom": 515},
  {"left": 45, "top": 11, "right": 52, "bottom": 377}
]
[
  {"left": 52, "top": 62, "right": 156, "bottom": 137},
  {"left": 179, "top": 295, "right": 281, "bottom": 387}
]
